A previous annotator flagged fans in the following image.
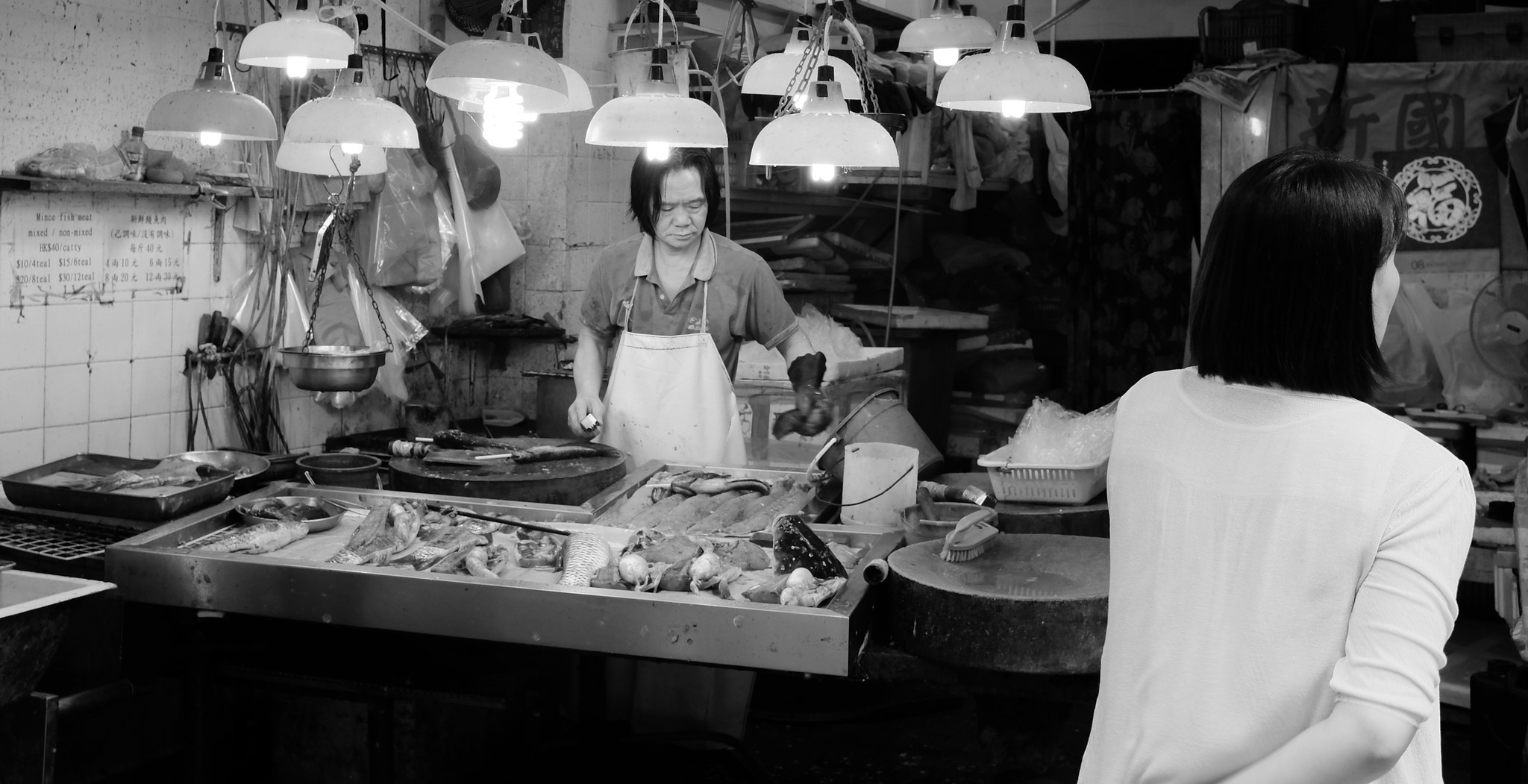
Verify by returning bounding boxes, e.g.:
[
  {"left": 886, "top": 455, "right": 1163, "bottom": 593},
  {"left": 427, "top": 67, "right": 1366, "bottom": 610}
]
[{"left": 1469, "top": 271, "right": 1528, "bottom": 424}]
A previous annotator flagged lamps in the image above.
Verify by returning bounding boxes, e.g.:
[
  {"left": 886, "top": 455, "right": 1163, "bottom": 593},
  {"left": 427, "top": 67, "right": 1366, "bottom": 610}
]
[
  {"left": 935, "top": 0, "right": 1092, "bottom": 118},
  {"left": 424, "top": 11, "right": 568, "bottom": 148},
  {"left": 749, "top": 64, "right": 900, "bottom": 181},
  {"left": 142, "top": 47, "right": 278, "bottom": 147},
  {"left": 457, "top": 0, "right": 594, "bottom": 140},
  {"left": 274, "top": 142, "right": 388, "bottom": 176},
  {"left": 741, "top": 0, "right": 863, "bottom": 110},
  {"left": 896, "top": 0, "right": 997, "bottom": 66},
  {"left": 584, "top": 0, "right": 729, "bottom": 161},
  {"left": 238, "top": 0, "right": 356, "bottom": 79},
  {"left": 284, "top": 54, "right": 421, "bottom": 150}
]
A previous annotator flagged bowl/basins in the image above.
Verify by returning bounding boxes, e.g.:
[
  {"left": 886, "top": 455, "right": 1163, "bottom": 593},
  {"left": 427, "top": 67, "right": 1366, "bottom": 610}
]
[{"left": 859, "top": 114, "right": 906, "bottom": 144}]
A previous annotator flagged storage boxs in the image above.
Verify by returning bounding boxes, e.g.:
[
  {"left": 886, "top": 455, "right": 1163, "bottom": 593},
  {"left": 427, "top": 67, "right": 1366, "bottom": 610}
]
[
  {"left": 734, "top": 370, "right": 909, "bottom": 473},
  {"left": 711, "top": 139, "right": 800, "bottom": 194},
  {"left": 978, "top": 445, "right": 1110, "bottom": 504},
  {"left": 1414, "top": 10, "right": 1528, "bottom": 60},
  {"left": 1207, "top": 3, "right": 1312, "bottom": 66}
]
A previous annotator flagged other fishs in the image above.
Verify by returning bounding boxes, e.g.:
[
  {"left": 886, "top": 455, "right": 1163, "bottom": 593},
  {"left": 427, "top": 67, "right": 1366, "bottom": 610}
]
[
  {"left": 189, "top": 519, "right": 308, "bottom": 558},
  {"left": 327, "top": 497, "right": 522, "bottom": 581},
  {"left": 620, "top": 473, "right": 816, "bottom": 595},
  {"left": 557, "top": 526, "right": 612, "bottom": 591},
  {"left": 511, "top": 447, "right": 615, "bottom": 463},
  {"left": 433, "top": 429, "right": 523, "bottom": 451}
]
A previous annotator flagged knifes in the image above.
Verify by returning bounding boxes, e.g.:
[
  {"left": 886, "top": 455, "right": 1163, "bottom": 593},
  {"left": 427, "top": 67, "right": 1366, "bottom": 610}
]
[{"left": 388, "top": 439, "right": 508, "bottom": 466}]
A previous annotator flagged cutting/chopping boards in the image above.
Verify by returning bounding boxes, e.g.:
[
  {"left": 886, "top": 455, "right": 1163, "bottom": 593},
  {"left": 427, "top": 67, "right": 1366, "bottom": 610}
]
[
  {"left": 888, "top": 472, "right": 1110, "bottom": 674},
  {"left": 385, "top": 436, "right": 627, "bottom": 504}
]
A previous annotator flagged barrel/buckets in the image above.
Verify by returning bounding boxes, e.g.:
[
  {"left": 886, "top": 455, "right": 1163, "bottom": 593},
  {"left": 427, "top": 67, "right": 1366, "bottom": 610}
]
[
  {"left": 805, "top": 388, "right": 943, "bottom": 526},
  {"left": 297, "top": 453, "right": 382, "bottom": 491},
  {"left": 607, "top": 0, "right": 693, "bottom": 99},
  {"left": 261, "top": 451, "right": 309, "bottom": 480}
]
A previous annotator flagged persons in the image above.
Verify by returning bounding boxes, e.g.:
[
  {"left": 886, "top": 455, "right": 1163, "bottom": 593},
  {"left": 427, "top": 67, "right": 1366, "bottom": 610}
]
[
  {"left": 1076, "top": 149, "right": 1479, "bottom": 784},
  {"left": 567, "top": 147, "right": 833, "bottom": 752}
]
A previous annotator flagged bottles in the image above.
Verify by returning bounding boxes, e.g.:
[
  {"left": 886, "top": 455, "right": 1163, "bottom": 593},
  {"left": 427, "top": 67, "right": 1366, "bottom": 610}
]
[{"left": 121, "top": 126, "right": 148, "bottom": 182}]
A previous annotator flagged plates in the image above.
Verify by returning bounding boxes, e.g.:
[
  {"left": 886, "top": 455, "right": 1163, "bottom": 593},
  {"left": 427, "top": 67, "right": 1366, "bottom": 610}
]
[
  {"left": 234, "top": 495, "right": 347, "bottom": 532},
  {"left": 162, "top": 450, "right": 271, "bottom": 483},
  {"left": 1471, "top": 492, "right": 1516, "bottom": 550}
]
